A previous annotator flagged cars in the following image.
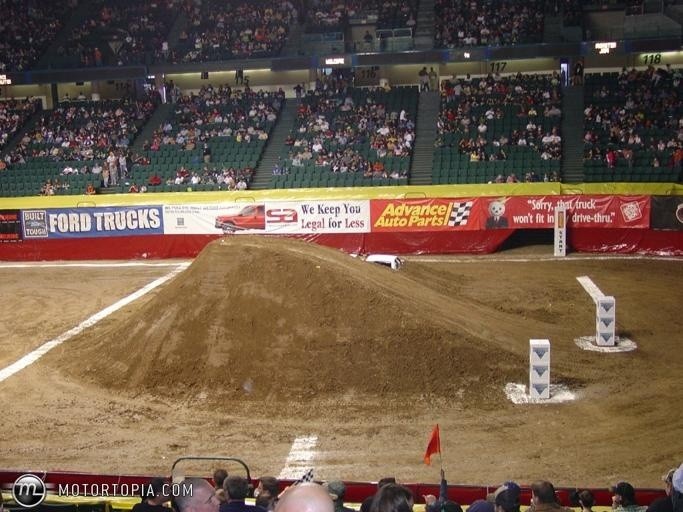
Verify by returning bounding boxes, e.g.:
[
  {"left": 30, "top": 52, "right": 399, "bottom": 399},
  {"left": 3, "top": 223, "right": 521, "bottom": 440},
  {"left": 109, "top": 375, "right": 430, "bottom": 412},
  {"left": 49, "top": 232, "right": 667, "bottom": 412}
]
[{"left": 216, "top": 204, "right": 265, "bottom": 234}]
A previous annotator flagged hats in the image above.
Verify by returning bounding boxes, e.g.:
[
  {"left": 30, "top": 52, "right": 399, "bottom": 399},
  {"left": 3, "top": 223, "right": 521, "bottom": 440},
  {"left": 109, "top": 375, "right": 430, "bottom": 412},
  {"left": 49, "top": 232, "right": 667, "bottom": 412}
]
[
  {"left": 610, "top": 482, "right": 634, "bottom": 497},
  {"left": 660, "top": 463, "right": 683, "bottom": 494},
  {"left": 465, "top": 481, "right": 520, "bottom": 512}
]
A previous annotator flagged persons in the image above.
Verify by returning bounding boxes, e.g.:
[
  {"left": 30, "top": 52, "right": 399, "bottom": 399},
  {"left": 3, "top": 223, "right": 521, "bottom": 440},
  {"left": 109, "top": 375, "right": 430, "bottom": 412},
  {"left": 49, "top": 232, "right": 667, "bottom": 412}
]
[
  {"left": 0, "top": 0, "right": 682, "bottom": 196},
  {"left": 130, "top": 463, "right": 681, "bottom": 512}
]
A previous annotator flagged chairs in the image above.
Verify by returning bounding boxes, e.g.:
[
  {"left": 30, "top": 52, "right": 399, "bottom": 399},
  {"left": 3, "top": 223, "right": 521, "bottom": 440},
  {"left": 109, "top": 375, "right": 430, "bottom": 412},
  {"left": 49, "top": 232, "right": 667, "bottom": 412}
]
[{"left": 0, "top": 0, "right": 683, "bottom": 197}]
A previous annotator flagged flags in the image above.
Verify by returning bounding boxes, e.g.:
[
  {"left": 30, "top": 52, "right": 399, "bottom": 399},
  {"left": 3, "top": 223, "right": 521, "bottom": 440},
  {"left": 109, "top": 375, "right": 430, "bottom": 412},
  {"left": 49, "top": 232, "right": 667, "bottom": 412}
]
[{"left": 422, "top": 422, "right": 441, "bottom": 466}]
